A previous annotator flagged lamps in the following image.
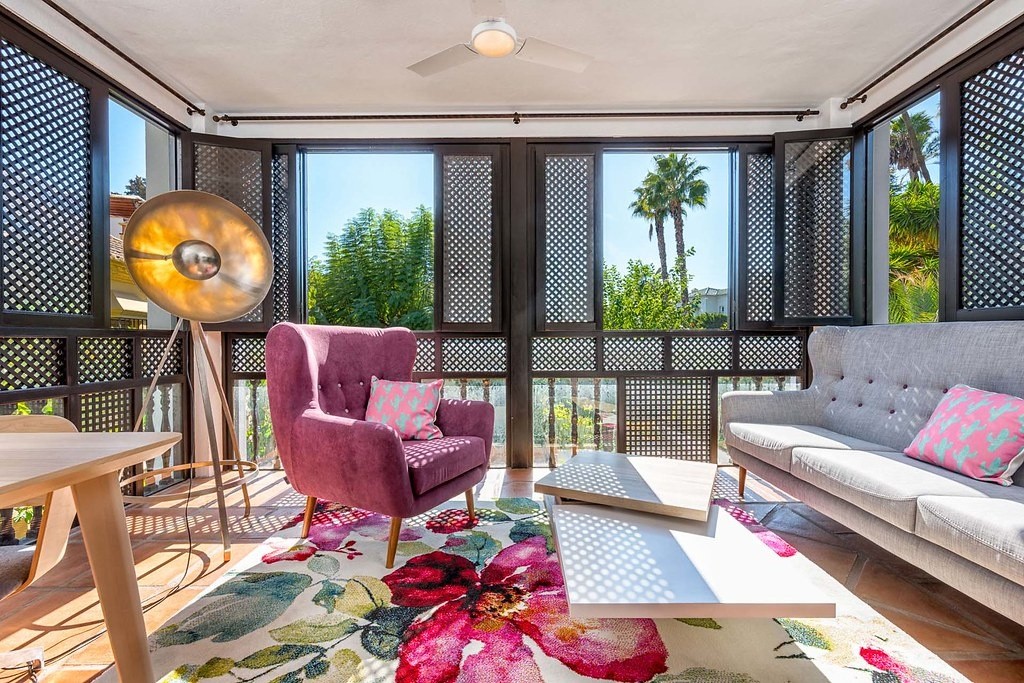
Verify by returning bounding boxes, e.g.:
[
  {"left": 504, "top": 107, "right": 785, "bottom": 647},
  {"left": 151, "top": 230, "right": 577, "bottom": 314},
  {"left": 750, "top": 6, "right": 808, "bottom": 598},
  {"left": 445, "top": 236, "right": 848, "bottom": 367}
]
[{"left": 474, "top": 30, "right": 516, "bottom": 57}]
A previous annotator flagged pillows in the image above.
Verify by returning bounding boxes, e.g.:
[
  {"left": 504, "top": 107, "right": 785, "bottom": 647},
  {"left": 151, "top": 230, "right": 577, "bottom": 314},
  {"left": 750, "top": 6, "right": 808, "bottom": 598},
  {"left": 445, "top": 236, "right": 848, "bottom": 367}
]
[
  {"left": 903, "top": 383, "right": 1024, "bottom": 488},
  {"left": 363, "top": 376, "right": 446, "bottom": 441}
]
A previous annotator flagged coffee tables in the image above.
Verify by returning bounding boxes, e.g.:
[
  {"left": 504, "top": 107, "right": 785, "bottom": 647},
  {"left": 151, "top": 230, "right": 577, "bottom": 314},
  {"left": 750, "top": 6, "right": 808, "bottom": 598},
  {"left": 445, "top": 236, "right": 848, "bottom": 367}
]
[{"left": 535, "top": 449, "right": 836, "bottom": 618}]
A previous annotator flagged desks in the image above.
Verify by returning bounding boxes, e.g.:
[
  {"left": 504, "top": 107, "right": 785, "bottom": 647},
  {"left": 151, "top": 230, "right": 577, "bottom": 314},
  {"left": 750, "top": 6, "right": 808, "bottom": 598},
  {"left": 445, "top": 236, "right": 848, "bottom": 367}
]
[{"left": 0, "top": 431, "right": 184, "bottom": 683}]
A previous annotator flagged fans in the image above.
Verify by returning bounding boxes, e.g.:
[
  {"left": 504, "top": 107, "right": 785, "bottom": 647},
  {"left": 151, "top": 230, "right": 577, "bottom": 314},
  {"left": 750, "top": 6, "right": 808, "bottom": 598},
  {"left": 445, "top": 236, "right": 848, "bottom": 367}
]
[{"left": 405, "top": 0, "right": 596, "bottom": 79}]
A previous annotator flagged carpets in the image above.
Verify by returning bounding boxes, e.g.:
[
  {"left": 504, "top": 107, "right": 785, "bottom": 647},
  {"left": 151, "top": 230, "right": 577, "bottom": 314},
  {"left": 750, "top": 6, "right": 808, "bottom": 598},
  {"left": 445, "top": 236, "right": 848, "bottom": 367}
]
[{"left": 90, "top": 496, "right": 972, "bottom": 683}]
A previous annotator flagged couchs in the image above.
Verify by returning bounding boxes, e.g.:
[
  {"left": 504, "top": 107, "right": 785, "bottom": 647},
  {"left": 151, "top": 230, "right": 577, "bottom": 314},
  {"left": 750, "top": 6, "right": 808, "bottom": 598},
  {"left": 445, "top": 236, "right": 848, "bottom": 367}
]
[
  {"left": 719, "top": 320, "right": 1024, "bottom": 627},
  {"left": 264, "top": 323, "right": 495, "bottom": 568}
]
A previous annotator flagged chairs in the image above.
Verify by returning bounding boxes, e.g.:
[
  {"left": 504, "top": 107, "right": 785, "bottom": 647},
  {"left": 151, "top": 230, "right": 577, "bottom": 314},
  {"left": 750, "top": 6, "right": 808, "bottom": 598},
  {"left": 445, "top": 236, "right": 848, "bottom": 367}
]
[{"left": 0, "top": 413, "right": 81, "bottom": 603}]
